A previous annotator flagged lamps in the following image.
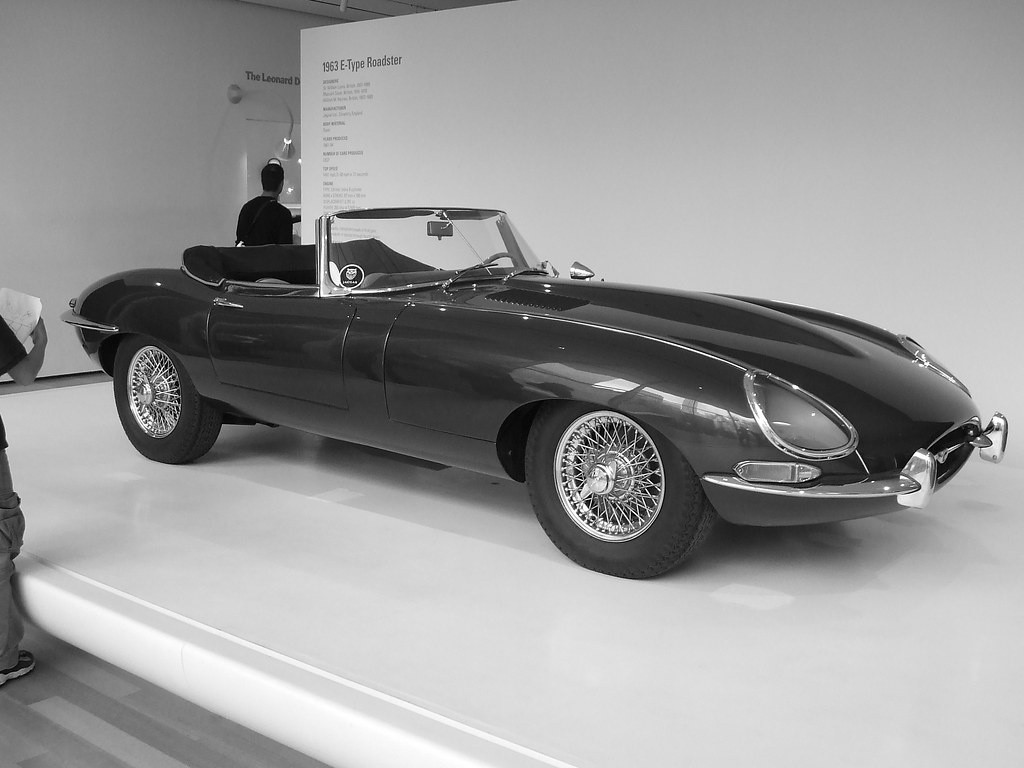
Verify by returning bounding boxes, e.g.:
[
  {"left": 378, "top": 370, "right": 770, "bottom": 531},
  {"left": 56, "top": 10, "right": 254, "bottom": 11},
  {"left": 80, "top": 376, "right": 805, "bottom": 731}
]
[{"left": 226, "top": 84, "right": 298, "bottom": 161}]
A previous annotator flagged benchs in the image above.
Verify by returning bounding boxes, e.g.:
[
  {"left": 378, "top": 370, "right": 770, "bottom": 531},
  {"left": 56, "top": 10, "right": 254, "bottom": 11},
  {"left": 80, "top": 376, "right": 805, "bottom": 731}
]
[{"left": 181, "top": 239, "right": 396, "bottom": 286}]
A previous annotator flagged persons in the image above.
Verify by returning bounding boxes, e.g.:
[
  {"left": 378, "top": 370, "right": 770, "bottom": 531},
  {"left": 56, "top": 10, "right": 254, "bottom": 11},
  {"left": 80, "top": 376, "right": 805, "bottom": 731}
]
[
  {"left": 0, "top": 285, "right": 47, "bottom": 686},
  {"left": 235, "top": 164, "right": 293, "bottom": 246}
]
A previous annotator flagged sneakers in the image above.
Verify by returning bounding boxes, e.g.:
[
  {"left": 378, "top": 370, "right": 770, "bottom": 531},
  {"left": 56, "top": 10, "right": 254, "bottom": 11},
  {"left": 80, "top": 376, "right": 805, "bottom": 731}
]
[{"left": 0, "top": 650, "right": 34, "bottom": 685}]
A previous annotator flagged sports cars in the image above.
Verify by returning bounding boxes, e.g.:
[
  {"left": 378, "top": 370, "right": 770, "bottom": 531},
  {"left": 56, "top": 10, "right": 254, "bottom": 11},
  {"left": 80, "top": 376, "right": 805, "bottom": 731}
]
[{"left": 56, "top": 204, "right": 1009, "bottom": 582}]
